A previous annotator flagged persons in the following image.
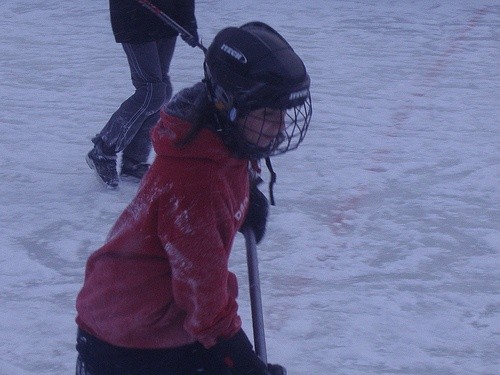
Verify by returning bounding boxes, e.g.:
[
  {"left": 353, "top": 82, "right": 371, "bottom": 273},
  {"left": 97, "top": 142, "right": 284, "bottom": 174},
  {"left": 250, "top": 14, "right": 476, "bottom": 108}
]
[
  {"left": 84, "top": 0, "right": 201, "bottom": 192},
  {"left": 74, "top": 21, "right": 313, "bottom": 375}
]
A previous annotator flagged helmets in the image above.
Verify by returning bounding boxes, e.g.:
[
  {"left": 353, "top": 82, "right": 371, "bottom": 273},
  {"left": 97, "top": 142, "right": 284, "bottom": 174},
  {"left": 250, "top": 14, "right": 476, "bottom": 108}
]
[{"left": 207, "top": 21, "right": 313, "bottom": 156}]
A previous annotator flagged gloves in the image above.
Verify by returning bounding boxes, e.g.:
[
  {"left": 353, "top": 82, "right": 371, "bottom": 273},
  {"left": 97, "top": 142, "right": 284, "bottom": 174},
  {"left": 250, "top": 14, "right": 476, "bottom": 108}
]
[
  {"left": 227, "top": 351, "right": 289, "bottom": 375},
  {"left": 242, "top": 185, "right": 271, "bottom": 244}
]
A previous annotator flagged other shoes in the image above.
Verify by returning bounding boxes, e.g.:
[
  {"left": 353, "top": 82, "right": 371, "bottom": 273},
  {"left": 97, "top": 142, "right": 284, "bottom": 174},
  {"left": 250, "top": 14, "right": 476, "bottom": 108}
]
[
  {"left": 86, "top": 150, "right": 120, "bottom": 188},
  {"left": 120, "top": 162, "right": 151, "bottom": 181}
]
